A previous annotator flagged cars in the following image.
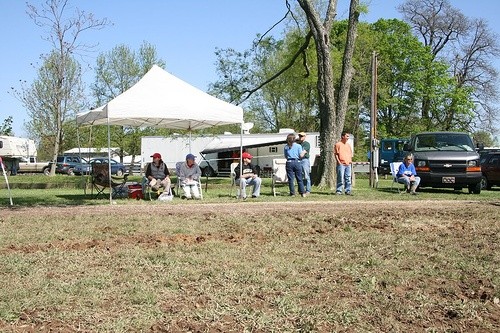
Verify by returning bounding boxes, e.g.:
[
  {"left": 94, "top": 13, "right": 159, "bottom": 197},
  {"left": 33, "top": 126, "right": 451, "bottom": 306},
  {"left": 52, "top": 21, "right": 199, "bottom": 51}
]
[
  {"left": 73, "top": 158, "right": 127, "bottom": 177},
  {"left": 479, "top": 151, "right": 500, "bottom": 190}
]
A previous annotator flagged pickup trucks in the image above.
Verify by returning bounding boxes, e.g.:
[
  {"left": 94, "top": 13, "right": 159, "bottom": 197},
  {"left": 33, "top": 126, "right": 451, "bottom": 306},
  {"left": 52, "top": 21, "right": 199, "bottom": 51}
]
[{"left": 10, "top": 155, "right": 50, "bottom": 173}]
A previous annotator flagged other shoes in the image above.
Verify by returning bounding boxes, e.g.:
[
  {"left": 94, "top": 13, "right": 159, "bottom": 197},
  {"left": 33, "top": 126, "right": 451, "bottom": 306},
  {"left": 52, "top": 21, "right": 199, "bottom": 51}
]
[
  {"left": 410, "top": 191, "right": 419, "bottom": 195},
  {"left": 301, "top": 194, "right": 306, "bottom": 197},
  {"left": 305, "top": 191, "right": 310, "bottom": 196},
  {"left": 345, "top": 192, "right": 355, "bottom": 196},
  {"left": 291, "top": 195, "right": 295, "bottom": 197},
  {"left": 337, "top": 192, "right": 343, "bottom": 195}
]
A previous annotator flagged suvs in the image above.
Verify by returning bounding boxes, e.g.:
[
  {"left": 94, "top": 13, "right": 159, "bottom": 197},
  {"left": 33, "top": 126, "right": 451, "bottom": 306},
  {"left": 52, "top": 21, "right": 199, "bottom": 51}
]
[
  {"left": 402, "top": 131, "right": 483, "bottom": 195},
  {"left": 48, "top": 155, "right": 88, "bottom": 175},
  {"left": 378, "top": 138, "right": 410, "bottom": 174}
]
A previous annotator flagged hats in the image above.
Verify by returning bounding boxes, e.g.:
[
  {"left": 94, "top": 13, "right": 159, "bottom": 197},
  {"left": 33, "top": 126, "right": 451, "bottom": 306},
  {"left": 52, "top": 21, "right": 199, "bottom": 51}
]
[
  {"left": 298, "top": 132, "right": 306, "bottom": 137},
  {"left": 242, "top": 152, "right": 253, "bottom": 158},
  {"left": 150, "top": 153, "right": 161, "bottom": 158},
  {"left": 186, "top": 154, "right": 197, "bottom": 160}
]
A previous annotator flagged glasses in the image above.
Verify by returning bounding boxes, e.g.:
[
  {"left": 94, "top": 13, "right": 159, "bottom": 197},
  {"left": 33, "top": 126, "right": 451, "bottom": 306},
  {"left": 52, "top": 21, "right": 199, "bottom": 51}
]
[{"left": 407, "top": 159, "right": 412, "bottom": 160}]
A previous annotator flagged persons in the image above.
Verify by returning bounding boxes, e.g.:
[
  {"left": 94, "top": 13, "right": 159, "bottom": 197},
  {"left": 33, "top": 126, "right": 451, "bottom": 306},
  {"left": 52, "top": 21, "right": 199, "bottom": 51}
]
[
  {"left": 284, "top": 132, "right": 311, "bottom": 198},
  {"left": 145, "top": 152, "right": 171, "bottom": 195},
  {"left": 234, "top": 152, "right": 262, "bottom": 198},
  {"left": 232, "top": 151, "right": 239, "bottom": 162},
  {"left": 394, "top": 155, "right": 421, "bottom": 195},
  {"left": 180, "top": 154, "right": 202, "bottom": 199},
  {"left": 333, "top": 131, "right": 353, "bottom": 195}
]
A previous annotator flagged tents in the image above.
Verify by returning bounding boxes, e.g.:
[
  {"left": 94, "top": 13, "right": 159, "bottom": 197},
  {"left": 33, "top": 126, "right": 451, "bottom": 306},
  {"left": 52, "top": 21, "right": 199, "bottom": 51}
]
[{"left": 74, "top": 63, "right": 244, "bottom": 204}]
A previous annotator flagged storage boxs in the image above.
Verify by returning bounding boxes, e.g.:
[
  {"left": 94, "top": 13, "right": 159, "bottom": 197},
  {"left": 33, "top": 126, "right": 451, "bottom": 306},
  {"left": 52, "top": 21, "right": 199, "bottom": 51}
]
[{"left": 127, "top": 184, "right": 143, "bottom": 198}]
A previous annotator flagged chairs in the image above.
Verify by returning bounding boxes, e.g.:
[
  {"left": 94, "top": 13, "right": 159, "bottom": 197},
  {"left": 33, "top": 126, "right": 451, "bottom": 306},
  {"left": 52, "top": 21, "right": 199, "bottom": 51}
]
[
  {"left": 176, "top": 162, "right": 203, "bottom": 200},
  {"left": 389, "top": 162, "right": 403, "bottom": 194},
  {"left": 91, "top": 161, "right": 129, "bottom": 199},
  {"left": 272, "top": 159, "right": 289, "bottom": 196},
  {"left": 142, "top": 162, "right": 173, "bottom": 202},
  {"left": 229, "top": 163, "right": 249, "bottom": 199}
]
